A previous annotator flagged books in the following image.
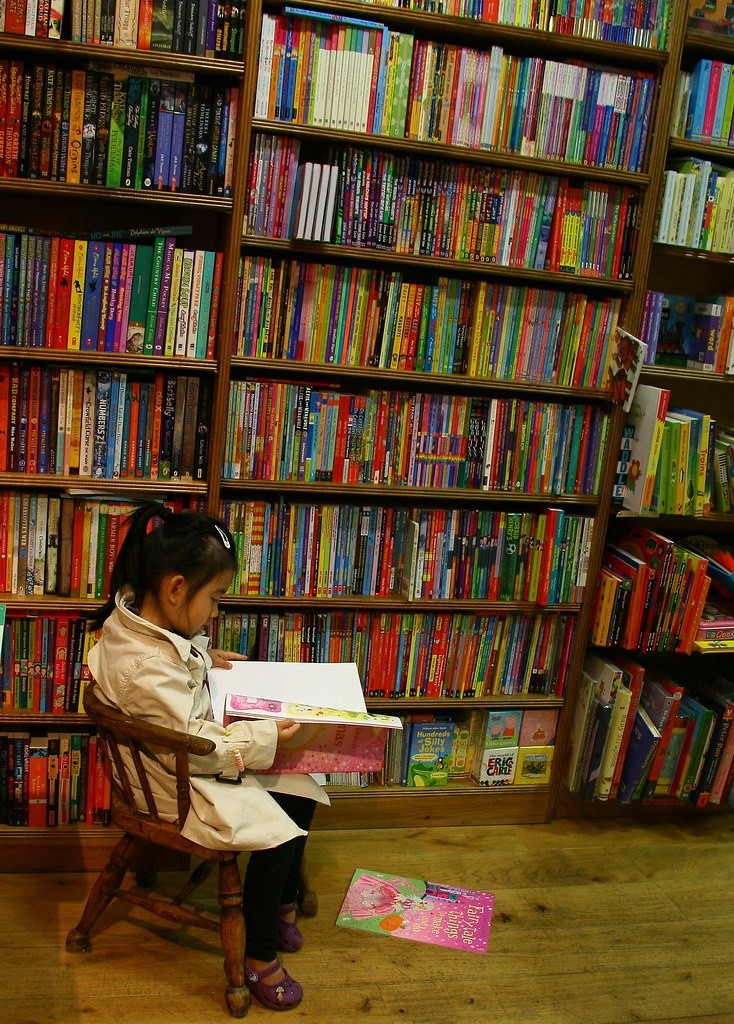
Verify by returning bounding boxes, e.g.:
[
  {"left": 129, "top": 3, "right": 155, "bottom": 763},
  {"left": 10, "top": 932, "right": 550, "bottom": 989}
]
[
  {"left": 0, "top": 0, "right": 732, "bottom": 827},
  {"left": 334, "top": 867, "right": 494, "bottom": 958}
]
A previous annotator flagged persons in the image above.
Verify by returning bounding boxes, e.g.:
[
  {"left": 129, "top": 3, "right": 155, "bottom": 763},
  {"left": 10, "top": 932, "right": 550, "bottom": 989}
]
[{"left": 86, "top": 495, "right": 319, "bottom": 1006}]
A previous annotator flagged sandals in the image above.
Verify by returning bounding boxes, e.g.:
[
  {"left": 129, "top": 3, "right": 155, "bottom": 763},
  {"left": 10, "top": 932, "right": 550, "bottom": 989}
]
[{"left": 245, "top": 902, "right": 303, "bottom": 1011}]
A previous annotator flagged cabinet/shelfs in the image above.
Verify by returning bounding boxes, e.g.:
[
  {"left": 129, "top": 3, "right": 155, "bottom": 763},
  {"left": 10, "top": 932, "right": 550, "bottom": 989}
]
[
  {"left": 215, "top": 0, "right": 693, "bottom": 831},
  {"left": 1, "top": 0, "right": 262, "bottom": 875},
  {"left": 553, "top": 0, "right": 734, "bottom": 821}
]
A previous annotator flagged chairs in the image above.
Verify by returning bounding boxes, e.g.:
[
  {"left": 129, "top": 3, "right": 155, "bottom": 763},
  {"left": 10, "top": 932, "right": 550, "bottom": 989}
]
[{"left": 64, "top": 681, "right": 319, "bottom": 1020}]
